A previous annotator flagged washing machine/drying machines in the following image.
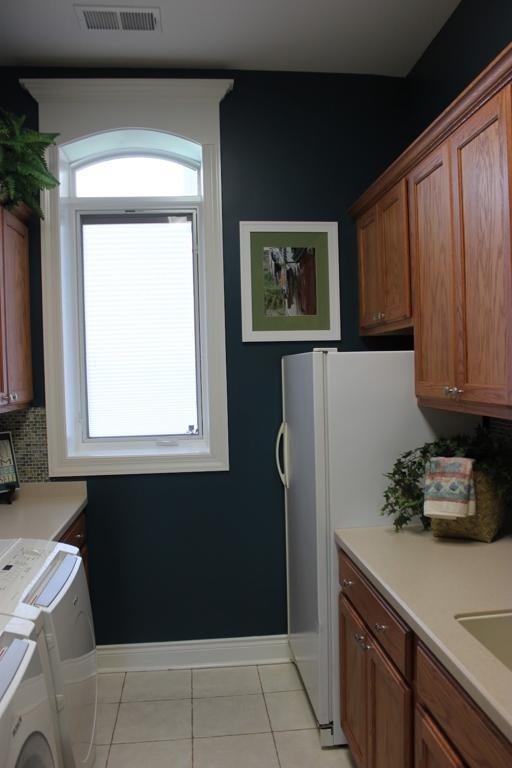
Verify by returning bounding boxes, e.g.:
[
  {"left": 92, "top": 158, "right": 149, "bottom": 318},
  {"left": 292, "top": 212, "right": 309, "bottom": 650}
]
[
  {"left": 0, "top": 613, "right": 64, "bottom": 768},
  {"left": 1, "top": 538, "right": 98, "bottom": 768}
]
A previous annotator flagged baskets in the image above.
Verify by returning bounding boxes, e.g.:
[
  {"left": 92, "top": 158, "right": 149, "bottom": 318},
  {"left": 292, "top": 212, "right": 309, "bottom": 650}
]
[{"left": 430, "top": 467, "right": 511, "bottom": 543}]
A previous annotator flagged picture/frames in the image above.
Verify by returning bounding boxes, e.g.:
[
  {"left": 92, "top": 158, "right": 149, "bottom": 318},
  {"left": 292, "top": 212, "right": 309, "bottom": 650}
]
[{"left": 239, "top": 220, "right": 342, "bottom": 342}]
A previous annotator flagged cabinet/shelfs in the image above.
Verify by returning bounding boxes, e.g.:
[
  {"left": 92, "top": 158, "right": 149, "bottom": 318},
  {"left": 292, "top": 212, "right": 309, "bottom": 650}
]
[
  {"left": 337, "top": 541, "right": 512, "bottom": 768},
  {"left": 412, "top": 37, "right": 512, "bottom": 419},
  {"left": 58, "top": 513, "right": 89, "bottom": 583},
  {"left": 349, "top": 148, "right": 410, "bottom": 338},
  {"left": 1, "top": 198, "right": 34, "bottom": 414}
]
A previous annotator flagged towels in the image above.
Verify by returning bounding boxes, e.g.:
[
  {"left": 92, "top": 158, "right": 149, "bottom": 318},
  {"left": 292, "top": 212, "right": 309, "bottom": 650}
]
[{"left": 422, "top": 457, "right": 479, "bottom": 520}]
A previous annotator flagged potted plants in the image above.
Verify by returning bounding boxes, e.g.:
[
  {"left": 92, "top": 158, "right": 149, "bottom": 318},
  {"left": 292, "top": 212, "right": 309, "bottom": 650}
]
[
  {"left": 378, "top": 430, "right": 511, "bottom": 542},
  {"left": 0, "top": 109, "right": 62, "bottom": 230}
]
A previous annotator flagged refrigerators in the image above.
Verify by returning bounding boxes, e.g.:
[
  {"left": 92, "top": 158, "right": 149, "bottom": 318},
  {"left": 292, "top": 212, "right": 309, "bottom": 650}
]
[{"left": 272, "top": 347, "right": 488, "bottom": 750}]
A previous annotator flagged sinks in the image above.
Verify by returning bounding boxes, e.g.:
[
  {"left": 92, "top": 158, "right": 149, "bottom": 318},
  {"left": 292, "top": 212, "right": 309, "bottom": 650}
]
[{"left": 453, "top": 609, "right": 512, "bottom": 671}]
